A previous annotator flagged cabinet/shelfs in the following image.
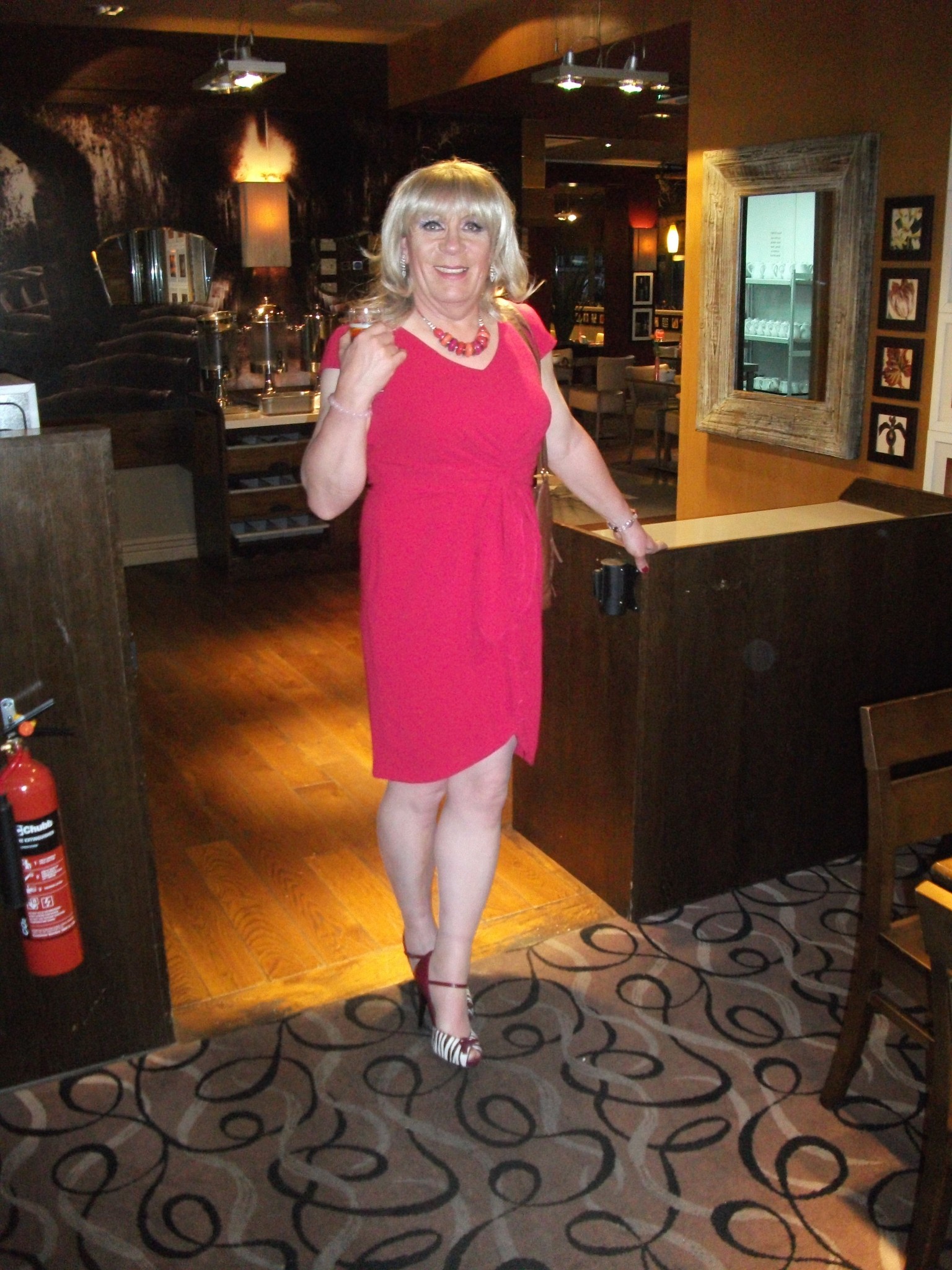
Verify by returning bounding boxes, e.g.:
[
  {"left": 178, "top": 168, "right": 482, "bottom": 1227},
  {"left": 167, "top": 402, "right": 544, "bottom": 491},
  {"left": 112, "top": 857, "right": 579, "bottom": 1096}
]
[{"left": 224, "top": 406, "right": 339, "bottom": 569}]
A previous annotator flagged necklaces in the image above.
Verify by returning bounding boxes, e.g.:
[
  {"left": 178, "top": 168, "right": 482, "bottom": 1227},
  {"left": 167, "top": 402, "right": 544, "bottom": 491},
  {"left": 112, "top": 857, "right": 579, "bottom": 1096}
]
[{"left": 416, "top": 308, "right": 491, "bottom": 357}]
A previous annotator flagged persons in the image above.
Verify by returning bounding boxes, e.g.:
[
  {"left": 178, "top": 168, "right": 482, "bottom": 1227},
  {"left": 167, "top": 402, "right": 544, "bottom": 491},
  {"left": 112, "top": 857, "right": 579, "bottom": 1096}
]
[{"left": 301, "top": 155, "right": 664, "bottom": 1068}]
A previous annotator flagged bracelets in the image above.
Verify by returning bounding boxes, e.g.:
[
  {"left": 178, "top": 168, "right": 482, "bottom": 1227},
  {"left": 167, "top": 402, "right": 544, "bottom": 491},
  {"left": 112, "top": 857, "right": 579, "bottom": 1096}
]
[
  {"left": 327, "top": 393, "right": 373, "bottom": 419},
  {"left": 607, "top": 509, "right": 638, "bottom": 532}
]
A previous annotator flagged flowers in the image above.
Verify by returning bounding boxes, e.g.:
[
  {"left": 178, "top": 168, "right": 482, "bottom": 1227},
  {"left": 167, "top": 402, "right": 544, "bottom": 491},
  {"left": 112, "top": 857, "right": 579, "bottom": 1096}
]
[{"left": 648, "top": 330, "right": 664, "bottom": 353}]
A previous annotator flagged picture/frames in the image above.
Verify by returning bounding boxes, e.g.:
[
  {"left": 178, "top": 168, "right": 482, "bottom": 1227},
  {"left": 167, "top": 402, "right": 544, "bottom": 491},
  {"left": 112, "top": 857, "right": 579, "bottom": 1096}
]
[
  {"left": 866, "top": 327, "right": 925, "bottom": 401},
  {"left": 854, "top": 394, "right": 923, "bottom": 472},
  {"left": 876, "top": 258, "right": 932, "bottom": 332},
  {"left": 872, "top": 192, "right": 936, "bottom": 266},
  {"left": 631, "top": 308, "right": 656, "bottom": 341},
  {"left": 696, "top": 127, "right": 880, "bottom": 462},
  {"left": 628, "top": 269, "right": 658, "bottom": 308}
]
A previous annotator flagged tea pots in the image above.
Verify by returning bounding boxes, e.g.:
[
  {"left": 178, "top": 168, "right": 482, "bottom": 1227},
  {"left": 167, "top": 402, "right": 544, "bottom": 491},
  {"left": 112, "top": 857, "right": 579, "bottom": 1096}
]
[
  {"left": 744, "top": 376, "right": 809, "bottom": 395},
  {"left": 744, "top": 317, "right": 811, "bottom": 340},
  {"left": 745, "top": 261, "right": 814, "bottom": 279}
]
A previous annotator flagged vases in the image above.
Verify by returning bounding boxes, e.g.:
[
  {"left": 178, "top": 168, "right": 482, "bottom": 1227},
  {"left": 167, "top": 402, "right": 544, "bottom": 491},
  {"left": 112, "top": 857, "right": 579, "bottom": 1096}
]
[{"left": 652, "top": 352, "right": 662, "bottom": 383}]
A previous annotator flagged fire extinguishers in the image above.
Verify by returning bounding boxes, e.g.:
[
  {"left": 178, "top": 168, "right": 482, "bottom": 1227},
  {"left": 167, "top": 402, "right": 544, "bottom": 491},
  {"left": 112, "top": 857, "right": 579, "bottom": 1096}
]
[{"left": 0, "top": 697, "right": 83, "bottom": 977}]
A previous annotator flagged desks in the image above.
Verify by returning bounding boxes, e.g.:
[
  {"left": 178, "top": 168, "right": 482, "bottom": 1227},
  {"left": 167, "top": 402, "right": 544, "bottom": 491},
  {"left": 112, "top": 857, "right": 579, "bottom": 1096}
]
[
  {"left": 506, "top": 473, "right": 952, "bottom": 923},
  {"left": 0, "top": 419, "right": 180, "bottom": 1092}
]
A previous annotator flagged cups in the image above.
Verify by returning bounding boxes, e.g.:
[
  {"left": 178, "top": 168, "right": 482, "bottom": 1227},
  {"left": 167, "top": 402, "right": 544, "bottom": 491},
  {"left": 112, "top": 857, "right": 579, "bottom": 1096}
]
[
  {"left": 349, "top": 308, "right": 381, "bottom": 343},
  {"left": 667, "top": 369, "right": 677, "bottom": 380},
  {"left": 579, "top": 335, "right": 587, "bottom": 344},
  {"left": 660, "top": 369, "right": 667, "bottom": 382}
]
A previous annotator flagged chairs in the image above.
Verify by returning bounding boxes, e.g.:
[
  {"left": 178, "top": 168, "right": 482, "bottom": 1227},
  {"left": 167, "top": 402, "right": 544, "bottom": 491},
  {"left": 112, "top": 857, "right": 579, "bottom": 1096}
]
[
  {"left": 3, "top": 217, "right": 222, "bottom": 291},
  {"left": 816, "top": 687, "right": 952, "bottom": 1110},
  {"left": 1, "top": 291, "right": 222, "bottom": 573},
  {"left": 551, "top": 346, "right": 679, "bottom": 468}
]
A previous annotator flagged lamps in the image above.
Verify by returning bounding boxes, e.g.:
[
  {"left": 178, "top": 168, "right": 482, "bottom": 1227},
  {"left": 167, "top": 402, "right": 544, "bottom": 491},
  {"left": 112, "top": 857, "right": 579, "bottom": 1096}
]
[
  {"left": 660, "top": 219, "right": 683, "bottom": 257},
  {"left": 226, "top": 172, "right": 305, "bottom": 279},
  {"left": 533, "top": 47, "right": 671, "bottom": 102},
  {"left": 547, "top": 180, "right": 584, "bottom": 224},
  {"left": 192, "top": 44, "right": 285, "bottom": 96}
]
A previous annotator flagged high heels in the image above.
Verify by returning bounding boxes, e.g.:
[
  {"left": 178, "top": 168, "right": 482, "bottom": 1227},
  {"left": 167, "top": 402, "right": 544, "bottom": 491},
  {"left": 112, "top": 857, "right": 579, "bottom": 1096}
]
[
  {"left": 402, "top": 929, "right": 476, "bottom": 1021},
  {"left": 412, "top": 951, "right": 482, "bottom": 1068}
]
